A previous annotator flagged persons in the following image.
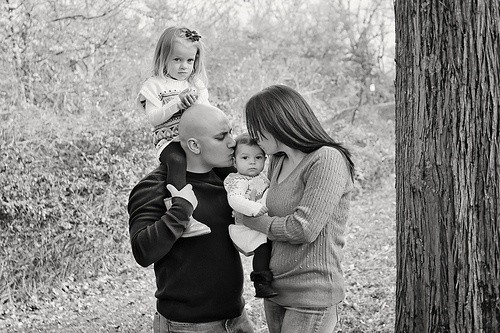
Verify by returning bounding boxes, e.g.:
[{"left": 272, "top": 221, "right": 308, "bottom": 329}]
[
  {"left": 223, "top": 133, "right": 279, "bottom": 299},
  {"left": 137, "top": 24, "right": 212, "bottom": 238},
  {"left": 127, "top": 103, "right": 254, "bottom": 333},
  {"left": 232, "top": 84, "right": 357, "bottom": 333}
]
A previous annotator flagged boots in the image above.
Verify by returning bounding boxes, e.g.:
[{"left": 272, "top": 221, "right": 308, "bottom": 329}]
[{"left": 163, "top": 197, "right": 211, "bottom": 238}]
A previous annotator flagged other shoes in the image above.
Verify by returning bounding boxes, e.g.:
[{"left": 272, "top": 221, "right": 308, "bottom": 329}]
[{"left": 255, "top": 280, "right": 279, "bottom": 298}]
